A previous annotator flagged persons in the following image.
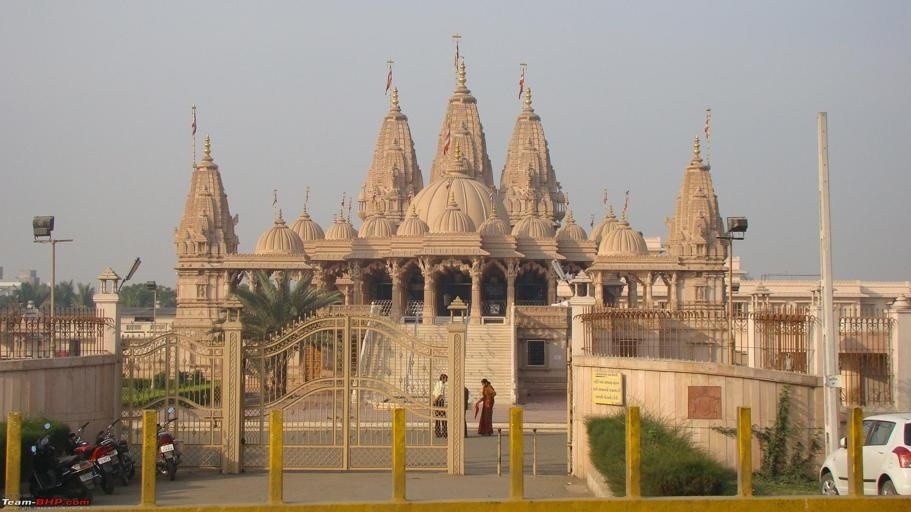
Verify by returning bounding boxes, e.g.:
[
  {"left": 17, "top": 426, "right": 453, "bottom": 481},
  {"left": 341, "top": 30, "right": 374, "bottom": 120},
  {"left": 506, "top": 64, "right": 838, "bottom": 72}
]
[
  {"left": 476, "top": 379, "right": 496, "bottom": 436},
  {"left": 432, "top": 374, "right": 448, "bottom": 439},
  {"left": 465, "top": 387, "right": 469, "bottom": 436}
]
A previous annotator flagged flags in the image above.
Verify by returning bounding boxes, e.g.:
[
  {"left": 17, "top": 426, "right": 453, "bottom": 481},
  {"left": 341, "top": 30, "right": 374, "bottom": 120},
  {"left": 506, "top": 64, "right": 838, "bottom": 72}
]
[
  {"left": 518, "top": 68, "right": 524, "bottom": 99},
  {"left": 385, "top": 68, "right": 392, "bottom": 92},
  {"left": 444, "top": 129, "right": 450, "bottom": 154}
]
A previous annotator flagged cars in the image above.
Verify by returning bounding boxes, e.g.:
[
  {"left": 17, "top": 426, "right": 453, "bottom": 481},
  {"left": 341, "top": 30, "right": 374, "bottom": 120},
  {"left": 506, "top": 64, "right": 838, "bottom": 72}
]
[{"left": 819, "top": 413, "right": 911, "bottom": 496}]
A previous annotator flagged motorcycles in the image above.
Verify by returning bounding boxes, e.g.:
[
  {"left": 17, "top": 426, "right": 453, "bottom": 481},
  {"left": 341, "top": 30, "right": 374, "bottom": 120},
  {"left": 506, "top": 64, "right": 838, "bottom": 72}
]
[
  {"left": 29, "top": 418, "right": 136, "bottom": 504},
  {"left": 156, "top": 407, "right": 182, "bottom": 481}
]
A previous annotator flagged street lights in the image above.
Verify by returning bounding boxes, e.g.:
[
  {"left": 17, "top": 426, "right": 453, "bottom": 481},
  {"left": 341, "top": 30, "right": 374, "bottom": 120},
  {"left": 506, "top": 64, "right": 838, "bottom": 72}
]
[
  {"left": 146, "top": 281, "right": 157, "bottom": 391},
  {"left": 32, "top": 214, "right": 74, "bottom": 360},
  {"left": 715, "top": 217, "right": 748, "bottom": 364}
]
